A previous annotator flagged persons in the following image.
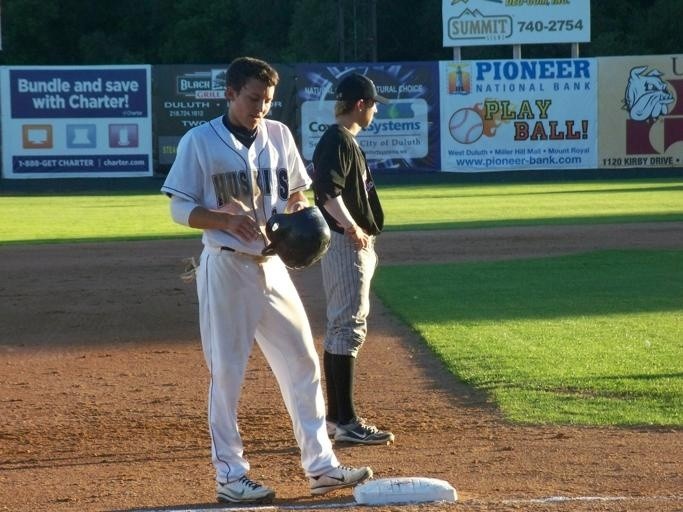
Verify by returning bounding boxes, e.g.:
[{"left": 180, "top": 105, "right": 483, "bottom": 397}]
[
  {"left": 309, "top": 71, "right": 395, "bottom": 446},
  {"left": 158, "top": 56, "right": 371, "bottom": 503}
]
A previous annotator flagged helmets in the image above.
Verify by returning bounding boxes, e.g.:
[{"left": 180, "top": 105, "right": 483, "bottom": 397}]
[{"left": 263, "top": 203, "right": 334, "bottom": 272}]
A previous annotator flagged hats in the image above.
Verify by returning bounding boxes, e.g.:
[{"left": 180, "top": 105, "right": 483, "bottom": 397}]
[{"left": 334, "top": 70, "right": 392, "bottom": 106}]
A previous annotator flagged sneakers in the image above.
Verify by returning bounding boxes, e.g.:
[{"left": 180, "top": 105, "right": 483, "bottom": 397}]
[
  {"left": 333, "top": 419, "right": 397, "bottom": 446},
  {"left": 324, "top": 415, "right": 338, "bottom": 440},
  {"left": 305, "top": 463, "right": 375, "bottom": 495},
  {"left": 213, "top": 474, "right": 277, "bottom": 505}
]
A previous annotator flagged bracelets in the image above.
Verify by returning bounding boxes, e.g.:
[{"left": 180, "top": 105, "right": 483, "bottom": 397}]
[{"left": 345, "top": 223, "right": 359, "bottom": 235}]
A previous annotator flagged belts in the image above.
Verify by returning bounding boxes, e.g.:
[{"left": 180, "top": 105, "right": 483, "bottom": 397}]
[{"left": 217, "top": 244, "right": 269, "bottom": 263}]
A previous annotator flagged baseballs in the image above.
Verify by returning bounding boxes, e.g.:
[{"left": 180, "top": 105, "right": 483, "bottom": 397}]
[{"left": 449, "top": 108, "right": 484, "bottom": 144}]
